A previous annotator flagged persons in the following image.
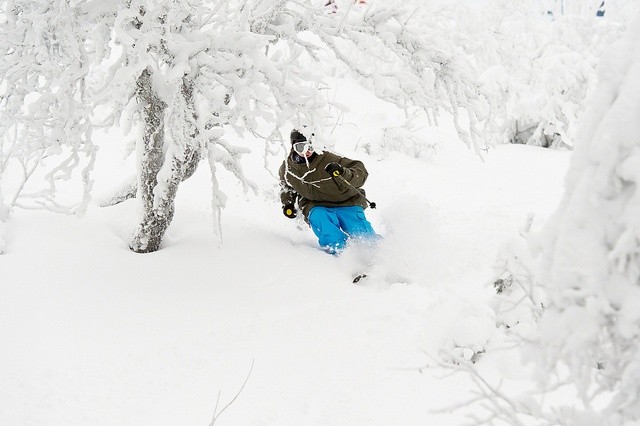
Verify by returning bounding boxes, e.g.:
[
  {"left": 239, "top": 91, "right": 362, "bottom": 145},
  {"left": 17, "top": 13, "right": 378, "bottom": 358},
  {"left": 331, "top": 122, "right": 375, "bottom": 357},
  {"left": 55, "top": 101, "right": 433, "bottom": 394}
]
[{"left": 279, "top": 126, "right": 382, "bottom": 257}]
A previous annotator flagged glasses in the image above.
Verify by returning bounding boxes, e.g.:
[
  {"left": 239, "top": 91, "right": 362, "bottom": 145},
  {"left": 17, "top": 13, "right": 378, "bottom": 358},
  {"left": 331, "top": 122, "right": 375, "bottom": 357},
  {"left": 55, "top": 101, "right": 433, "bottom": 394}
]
[{"left": 292, "top": 141, "right": 314, "bottom": 155}]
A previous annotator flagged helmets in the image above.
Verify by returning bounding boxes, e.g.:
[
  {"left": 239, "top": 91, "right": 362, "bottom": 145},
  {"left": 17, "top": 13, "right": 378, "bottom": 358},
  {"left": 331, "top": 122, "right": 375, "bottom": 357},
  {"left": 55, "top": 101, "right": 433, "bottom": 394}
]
[{"left": 290, "top": 124, "right": 315, "bottom": 144}]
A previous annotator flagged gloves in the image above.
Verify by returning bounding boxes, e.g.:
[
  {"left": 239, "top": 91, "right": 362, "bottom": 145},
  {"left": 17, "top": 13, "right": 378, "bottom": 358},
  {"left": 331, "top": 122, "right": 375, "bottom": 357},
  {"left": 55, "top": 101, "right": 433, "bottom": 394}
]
[
  {"left": 325, "top": 162, "right": 343, "bottom": 176},
  {"left": 283, "top": 203, "right": 297, "bottom": 219}
]
